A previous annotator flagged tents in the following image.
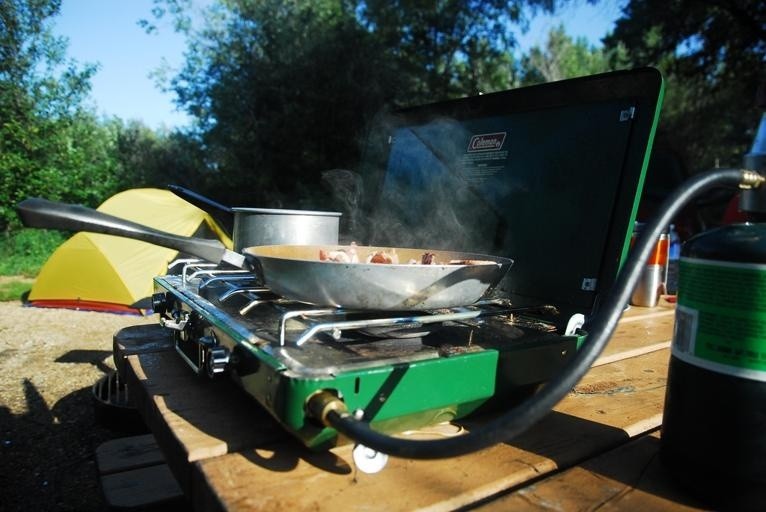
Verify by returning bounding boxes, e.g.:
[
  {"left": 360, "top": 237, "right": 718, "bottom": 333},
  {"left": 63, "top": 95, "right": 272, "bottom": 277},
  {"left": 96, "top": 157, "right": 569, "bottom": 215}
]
[{"left": 26, "top": 188, "right": 235, "bottom": 316}]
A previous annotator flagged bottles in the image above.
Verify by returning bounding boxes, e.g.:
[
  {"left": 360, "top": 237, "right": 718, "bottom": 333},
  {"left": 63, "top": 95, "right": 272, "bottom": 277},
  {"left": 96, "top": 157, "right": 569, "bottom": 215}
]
[
  {"left": 667, "top": 221, "right": 680, "bottom": 295},
  {"left": 630, "top": 220, "right": 669, "bottom": 310}
]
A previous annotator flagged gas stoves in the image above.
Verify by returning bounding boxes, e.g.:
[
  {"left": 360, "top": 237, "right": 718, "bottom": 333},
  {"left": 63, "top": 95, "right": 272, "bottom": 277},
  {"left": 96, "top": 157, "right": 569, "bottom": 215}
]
[{"left": 148, "top": 67, "right": 664, "bottom": 451}]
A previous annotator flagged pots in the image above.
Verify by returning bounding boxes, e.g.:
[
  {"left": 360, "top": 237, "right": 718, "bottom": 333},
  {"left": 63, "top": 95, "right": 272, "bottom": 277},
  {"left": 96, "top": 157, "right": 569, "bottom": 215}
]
[
  {"left": 167, "top": 181, "right": 342, "bottom": 272},
  {"left": 11, "top": 197, "right": 514, "bottom": 312}
]
